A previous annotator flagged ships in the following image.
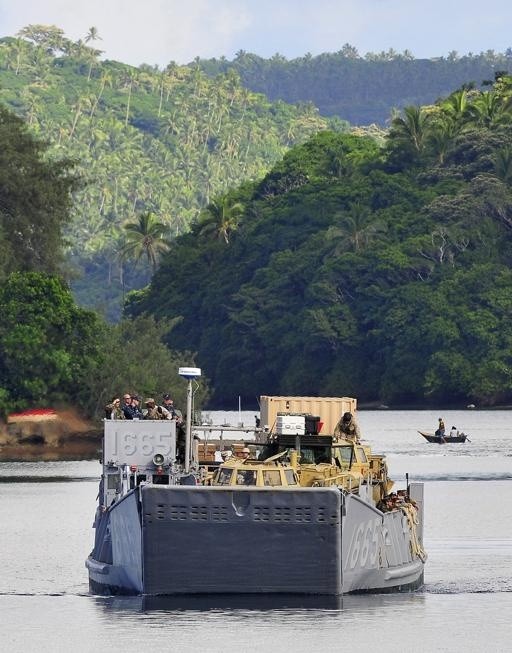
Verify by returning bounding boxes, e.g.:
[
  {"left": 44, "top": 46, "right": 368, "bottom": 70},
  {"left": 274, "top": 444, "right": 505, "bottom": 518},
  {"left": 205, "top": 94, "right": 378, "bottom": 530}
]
[{"left": 85, "top": 365, "right": 427, "bottom": 597}]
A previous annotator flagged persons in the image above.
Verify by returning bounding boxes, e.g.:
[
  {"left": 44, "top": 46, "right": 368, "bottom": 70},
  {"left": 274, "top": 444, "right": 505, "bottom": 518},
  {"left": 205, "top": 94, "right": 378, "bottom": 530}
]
[
  {"left": 314, "top": 447, "right": 329, "bottom": 465},
  {"left": 334, "top": 412, "right": 361, "bottom": 446},
  {"left": 213, "top": 447, "right": 233, "bottom": 465},
  {"left": 438, "top": 418, "right": 447, "bottom": 444},
  {"left": 299, "top": 452, "right": 313, "bottom": 465},
  {"left": 104, "top": 392, "right": 182, "bottom": 431},
  {"left": 434, "top": 429, "right": 446, "bottom": 437},
  {"left": 376, "top": 490, "right": 419, "bottom": 513},
  {"left": 449, "top": 426, "right": 458, "bottom": 437},
  {"left": 254, "top": 415, "right": 260, "bottom": 434},
  {"left": 280, "top": 453, "right": 290, "bottom": 463},
  {"left": 236, "top": 448, "right": 254, "bottom": 485}
]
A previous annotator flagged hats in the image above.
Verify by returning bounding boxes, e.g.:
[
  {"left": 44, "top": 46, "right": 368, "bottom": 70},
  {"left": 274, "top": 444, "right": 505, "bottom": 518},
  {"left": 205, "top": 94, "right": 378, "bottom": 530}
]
[
  {"left": 130, "top": 394, "right": 143, "bottom": 401},
  {"left": 144, "top": 398, "right": 154, "bottom": 403},
  {"left": 163, "top": 393, "right": 170, "bottom": 400},
  {"left": 342, "top": 412, "right": 351, "bottom": 422}
]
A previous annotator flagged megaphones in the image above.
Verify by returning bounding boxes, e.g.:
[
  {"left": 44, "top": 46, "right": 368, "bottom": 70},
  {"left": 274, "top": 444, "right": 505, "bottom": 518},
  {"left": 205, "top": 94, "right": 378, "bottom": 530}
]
[{"left": 153, "top": 454, "right": 165, "bottom": 466}]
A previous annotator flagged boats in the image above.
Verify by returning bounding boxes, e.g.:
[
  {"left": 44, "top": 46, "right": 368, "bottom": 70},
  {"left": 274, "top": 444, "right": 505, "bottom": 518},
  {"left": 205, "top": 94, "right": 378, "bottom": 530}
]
[{"left": 417, "top": 431, "right": 467, "bottom": 444}]
